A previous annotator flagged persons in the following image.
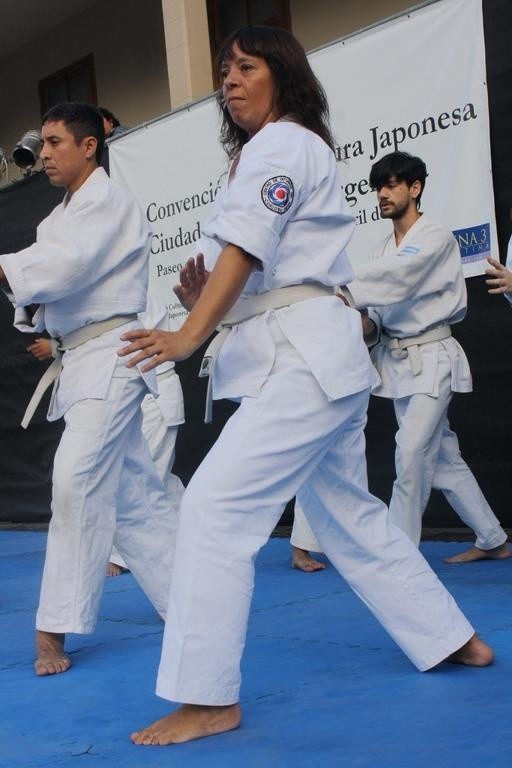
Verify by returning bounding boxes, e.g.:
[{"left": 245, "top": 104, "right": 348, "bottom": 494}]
[
  {"left": 485, "top": 228, "right": 512, "bottom": 308},
  {"left": 334, "top": 147, "right": 512, "bottom": 564},
  {"left": 26, "top": 297, "right": 187, "bottom": 578},
  {"left": 288, "top": 494, "right": 329, "bottom": 573},
  {"left": 118, "top": 18, "right": 495, "bottom": 749},
  {"left": 1, "top": 100, "right": 190, "bottom": 678},
  {"left": 98, "top": 105, "right": 130, "bottom": 139}
]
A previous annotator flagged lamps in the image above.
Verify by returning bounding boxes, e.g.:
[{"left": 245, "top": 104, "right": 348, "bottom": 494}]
[{"left": 13, "top": 129, "right": 45, "bottom": 171}]
